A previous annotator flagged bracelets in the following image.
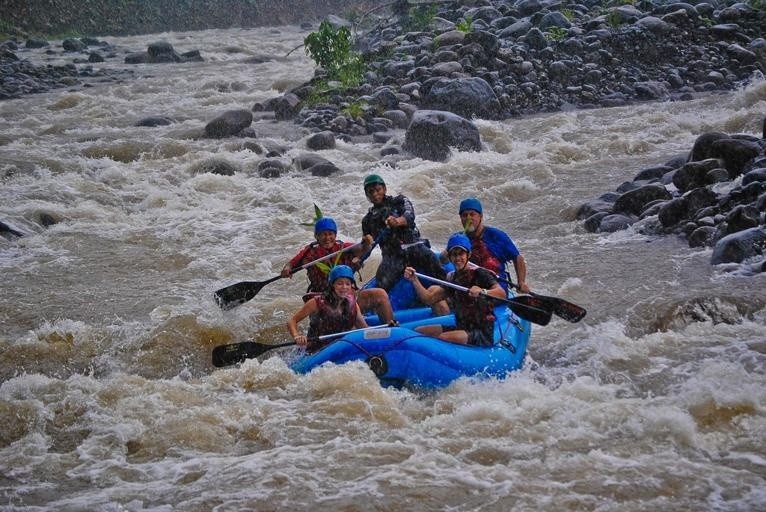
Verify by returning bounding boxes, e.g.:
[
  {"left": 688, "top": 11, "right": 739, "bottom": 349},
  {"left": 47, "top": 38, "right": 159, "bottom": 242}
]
[{"left": 480, "top": 289, "right": 487, "bottom": 300}]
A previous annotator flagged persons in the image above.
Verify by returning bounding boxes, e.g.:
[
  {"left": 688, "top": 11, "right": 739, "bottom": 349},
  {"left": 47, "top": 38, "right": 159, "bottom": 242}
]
[
  {"left": 432, "top": 198, "right": 529, "bottom": 316},
  {"left": 404, "top": 234, "right": 507, "bottom": 348},
  {"left": 352, "top": 174, "right": 447, "bottom": 293},
  {"left": 286, "top": 264, "right": 369, "bottom": 355},
  {"left": 281, "top": 217, "right": 394, "bottom": 325}
]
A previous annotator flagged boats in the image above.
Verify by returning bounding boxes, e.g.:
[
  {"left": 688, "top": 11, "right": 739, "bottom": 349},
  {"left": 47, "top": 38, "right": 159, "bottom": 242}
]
[{"left": 291, "top": 267, "right": 532, "bottom": 388}]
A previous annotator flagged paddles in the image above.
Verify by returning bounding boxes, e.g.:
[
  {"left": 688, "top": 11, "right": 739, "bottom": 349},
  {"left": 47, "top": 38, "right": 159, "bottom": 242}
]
[
  {"left": 214, "top": 240, "right": 364, "bottom": 311},
  {"left": 492, "top": 275, "right": 586, "bottom": 323},
  {"left": 411, "top": 270, "right": 553, "bottom": 326},
  {"left": 212, "top": 324, "right": 394, "bottom": 368}
]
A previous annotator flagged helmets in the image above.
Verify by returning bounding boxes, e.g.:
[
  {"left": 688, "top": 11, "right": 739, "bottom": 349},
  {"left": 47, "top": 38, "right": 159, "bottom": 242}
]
[
  {"left": 314, "top": 217, "right": 337, "bottom": 235},
  {"left": 447, "top": 234, "right": 472, "bottom": 252},
  {"left": 328, "top": 265, "right": 354, "bottom": 289},
  {"left": 459, "top": 198, "right": 483, "bottom": 215},
  {"left": 364, "top": 175, "right": 386, "bottom": 191}
]
